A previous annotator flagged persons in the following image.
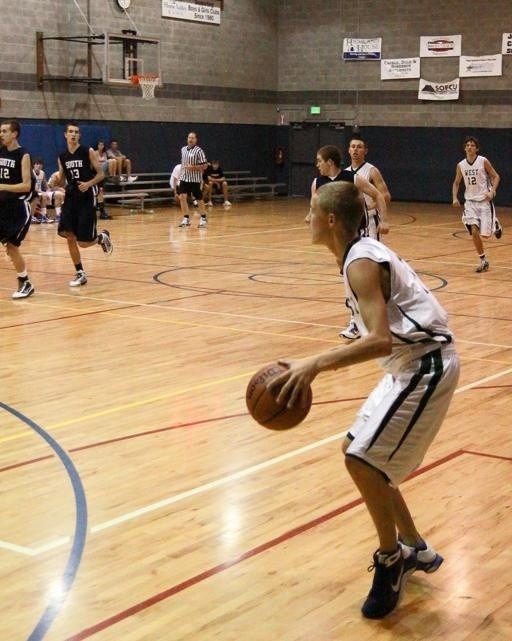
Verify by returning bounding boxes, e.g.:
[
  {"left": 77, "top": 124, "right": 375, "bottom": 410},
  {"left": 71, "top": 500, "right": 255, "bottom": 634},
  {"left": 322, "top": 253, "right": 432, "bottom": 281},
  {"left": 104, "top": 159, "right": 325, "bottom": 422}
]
[
  {"left": 201, "top": 161, "right": 212, "bottom": 201},
  {"left": 0, "top": 121, "right": 34, "bottom": 298},
  {"left": 342, "top": 139, "right": 391, "bottom": 241},
  {"left": 304, "top": 146, "right": 389, "bottom": 343},
  {"left": 94, "top": 140, "right": 119, "bottom": 182},
  {"left": 451, "top": 134, "right": 501, "bottom": 273},
  {"left": 169, "top": 164, "right": 198, "bottom": 207},
  {"left": 204, "top": 158, "right": 229, "bottom": 207},
  {"left": 107, "top": 139, "right": 136, "bottom": 181},
  {"left": 268, "top": 181, "right": 460, "bottom": 620},
  {"left": 48, "top": 172, "right": 70, "bottom": 221},
  {"left": 52, "top": 121, "right": 112, "bottom": 287},
  {"left": 178, "top": 133, "right": 208, "bottom": 228},
  {"left": 93, "top": 169, "right": 112, "bottom": 220},
  {"left": 30, "top": 157, "right": 54, "bottom": 223}
]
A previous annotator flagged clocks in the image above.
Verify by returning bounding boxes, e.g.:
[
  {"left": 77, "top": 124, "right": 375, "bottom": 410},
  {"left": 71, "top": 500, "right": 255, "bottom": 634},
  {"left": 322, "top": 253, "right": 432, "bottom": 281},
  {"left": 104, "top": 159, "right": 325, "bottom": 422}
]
[{"left": 118, "top": 0, "right": 130, "bottom": 8}]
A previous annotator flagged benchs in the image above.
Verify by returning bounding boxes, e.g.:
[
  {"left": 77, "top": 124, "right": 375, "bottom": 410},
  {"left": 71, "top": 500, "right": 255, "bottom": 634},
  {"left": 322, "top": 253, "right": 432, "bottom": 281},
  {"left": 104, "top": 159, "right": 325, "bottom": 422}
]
[
  {"left": 94, "top": 172, "right": 287, "bottom": 212},
  {"left": 97, "top": 192, "right": 148, "bottom": 212}
]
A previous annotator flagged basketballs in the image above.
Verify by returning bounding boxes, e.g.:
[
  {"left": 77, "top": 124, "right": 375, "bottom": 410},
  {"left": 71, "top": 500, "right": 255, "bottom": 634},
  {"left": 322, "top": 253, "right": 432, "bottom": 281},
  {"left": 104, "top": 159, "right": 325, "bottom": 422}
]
[{"left": 245, "top": 364, "right": 313, "bottom": 430}]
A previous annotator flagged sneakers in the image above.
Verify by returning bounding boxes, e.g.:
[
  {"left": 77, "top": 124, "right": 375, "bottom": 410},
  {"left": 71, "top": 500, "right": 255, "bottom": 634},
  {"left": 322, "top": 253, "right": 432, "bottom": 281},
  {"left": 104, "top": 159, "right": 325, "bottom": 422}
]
[
  {"left": 395, "top": 532, "right": 444, "bottom": 575},
  {"left": 10, "top": 279, "right": 35, "bottom": 300},
  {"left": 360, "top": 542, "right": 418, "bottom": 620},
  {"left": 99, "top": 229, "right": 113, "bottom": 257},
  {"left": 494, "top": 217, "right": 503, "bottom": 239},
  {"left": 198, "top": 217, "right": 208, "bottom": 228},
  {"left": 339, "top": 322, "right": 362, "bottom": 340},
  {"left": 30, "top": 215, "right": 41, "bottom": 224},
  {"left": 68, "top": 270, "right": 88, "bottom": 287},
  {"left": 222, "top": 200, "right": 232, "bottom": 206},
  {"left": 474, "top": 260, "right": 490, "bottom": 273},
  {"left": 177, "top": 222, "right": 192, "bottom": 230},
  {"left": 207, "top": 200, "right": 212, "bottom": 207},
  {"left": 99, "top": 213, "right": 112, "bottom": 219},
  {"left": 41, "top": 215, "right": 54, "bottom": 223}
]
